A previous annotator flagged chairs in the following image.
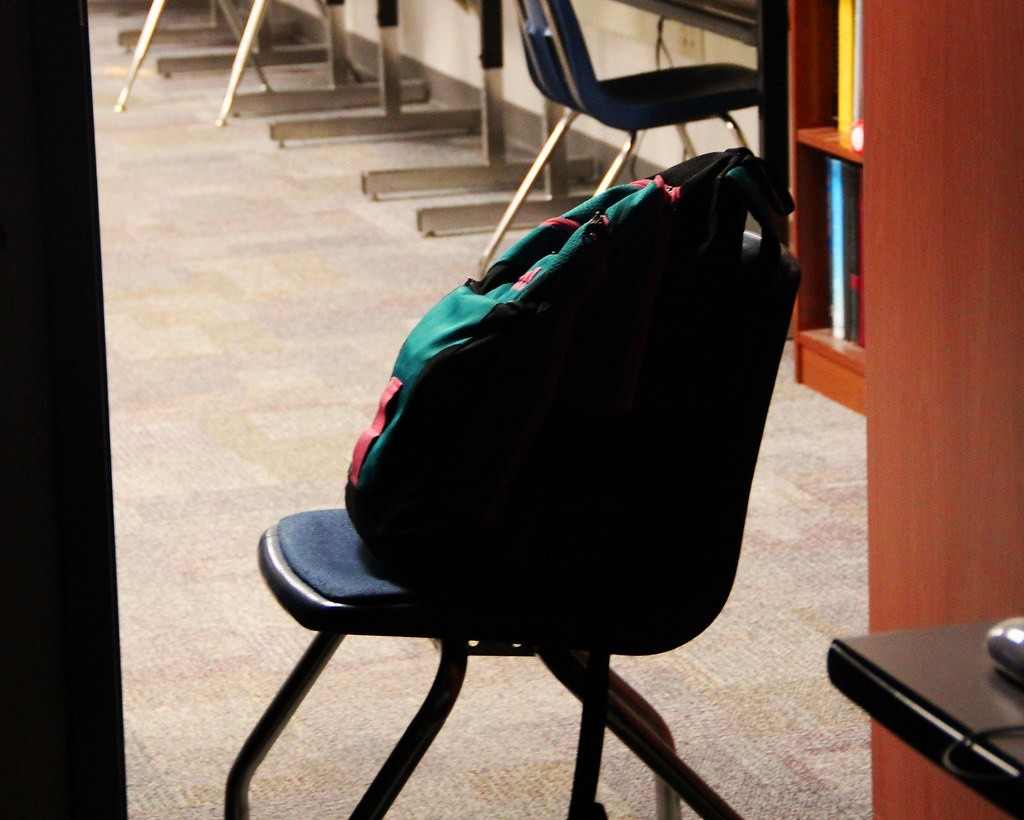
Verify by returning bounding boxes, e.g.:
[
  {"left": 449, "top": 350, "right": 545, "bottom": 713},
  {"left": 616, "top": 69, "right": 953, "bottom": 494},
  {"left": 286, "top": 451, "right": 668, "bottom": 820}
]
[
  {"left": 225, "top": 227, "right": 804, "bottom": 820},
  {"left": 476, "top": 0, "right": 764, "bottom": 283}
]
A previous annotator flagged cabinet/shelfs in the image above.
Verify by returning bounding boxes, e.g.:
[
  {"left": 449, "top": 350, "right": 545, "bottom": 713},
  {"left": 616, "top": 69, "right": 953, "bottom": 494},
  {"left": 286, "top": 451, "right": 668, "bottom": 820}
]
[{"left": 789, "top": 0, "right": 874, "bottom": 418}]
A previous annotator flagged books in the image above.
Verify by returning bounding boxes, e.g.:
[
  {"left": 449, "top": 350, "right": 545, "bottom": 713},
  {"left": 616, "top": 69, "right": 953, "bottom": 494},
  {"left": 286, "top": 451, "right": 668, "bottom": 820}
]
[
  {"left": 836, "top": 0, "right": 864, "bottom": 152},
  {"left": 827, "top": 153, "right": 864, "bottom": 351}
]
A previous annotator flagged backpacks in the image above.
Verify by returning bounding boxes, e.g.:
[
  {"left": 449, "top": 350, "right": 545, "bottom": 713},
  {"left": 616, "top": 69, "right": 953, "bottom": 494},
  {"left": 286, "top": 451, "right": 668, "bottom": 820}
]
[{"left": 345, "top": 144, "right": 795, "bottom": 597}]
